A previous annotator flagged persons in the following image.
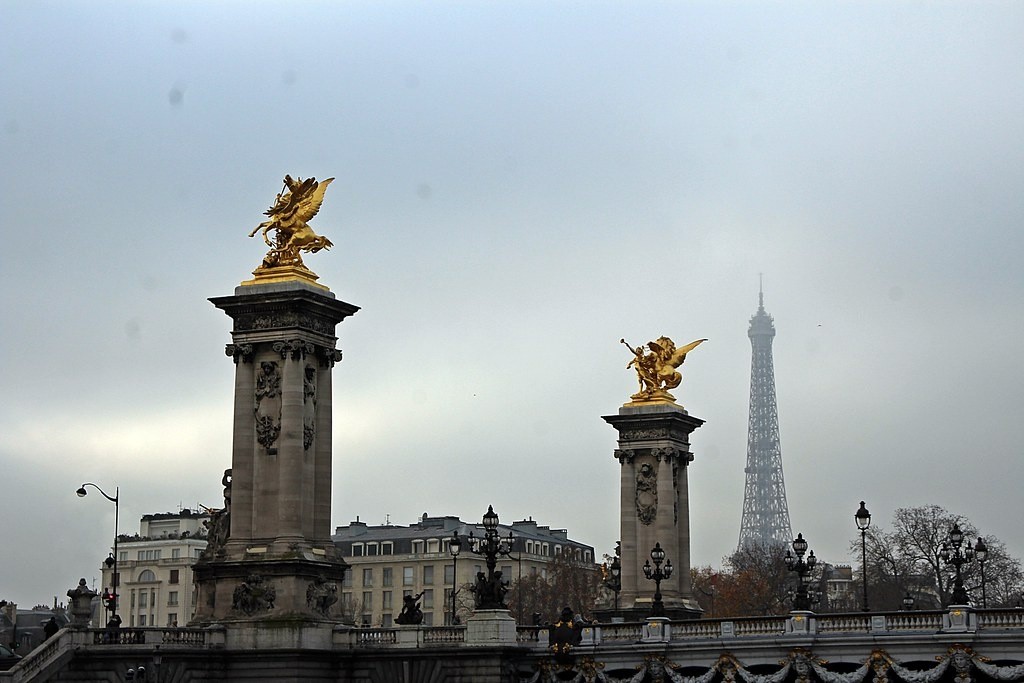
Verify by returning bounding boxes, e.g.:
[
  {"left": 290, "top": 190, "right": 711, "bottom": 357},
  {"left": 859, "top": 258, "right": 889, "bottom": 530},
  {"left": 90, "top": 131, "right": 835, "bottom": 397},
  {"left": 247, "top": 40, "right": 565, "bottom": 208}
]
[
  {"left": 402, "top": 591, "right": 425, "bottom": 614},
  {"left": 78, "top": 578, "right": 88, "bottom": 589},
  {"left": 169, "top": 620, "right": 178, "bottom": 628},
  {"left": 203, "top": 520, "right": 214, "bottom": 542},
  {"left": 362, "top": 620, "right": 370, "bottom": 627},
  {"left": 593, "top": 620, "right": 598, "bottom": 624},
  {"left": 44, "top": 616, "right": 60, "bottom": 641},
  {"left": 452, "top": 614, "right": 461, "bottom": 625},
  {"left": 574, "top": 614, "right": 582, "bottom": 622},
  {"left": 626, "top": 343, "right": 656, "bottom": 394}
]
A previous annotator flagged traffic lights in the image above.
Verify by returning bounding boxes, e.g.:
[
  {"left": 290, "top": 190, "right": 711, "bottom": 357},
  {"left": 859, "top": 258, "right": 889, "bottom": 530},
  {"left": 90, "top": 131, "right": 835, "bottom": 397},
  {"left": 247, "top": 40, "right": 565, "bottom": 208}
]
[
  {"left": 9, "top": 642, "right": 20, "bottom": 649},
  {"left": 534, "top": 612, "right": 541, "bottom": 625},
  {"left": 109, "top": 593, "right": 116, "bottom": 610}
]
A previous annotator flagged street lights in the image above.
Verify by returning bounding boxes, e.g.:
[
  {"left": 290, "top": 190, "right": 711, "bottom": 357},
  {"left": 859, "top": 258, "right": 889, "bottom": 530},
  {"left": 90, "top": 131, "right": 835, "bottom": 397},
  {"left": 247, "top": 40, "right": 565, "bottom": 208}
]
[
  {"left": 469, "top": 506, "right": 513, "bottom": 609},
  {"left": 854, "top": 501, "right": 872, "bottom": 612},
  {"left": 975, "top": 536, "right": 988, "bottom": 608},
  {"left": 449, "top": 530, "right": 461, "bottom": 624},
  {"left": 785, "top": 533, "right": 822, "bottom": 610},
  {"left": 611, "top": 557, "right": 621, "bottom": 617},
  {"left": 642, "top": 542, "right": 673, "bottom": 618},
  {"left": 76, "top": 482, "right": 120, "bottom": 628},
  {"left": 942, "top": 525, "right": 975, "bottom": 605}
]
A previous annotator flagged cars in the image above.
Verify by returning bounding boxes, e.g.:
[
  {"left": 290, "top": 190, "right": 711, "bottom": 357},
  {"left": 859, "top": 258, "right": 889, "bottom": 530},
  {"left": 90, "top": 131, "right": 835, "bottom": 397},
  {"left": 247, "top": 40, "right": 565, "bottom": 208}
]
[{"left": 0, "top": 644, "right": 23, "bottom": 670}]
